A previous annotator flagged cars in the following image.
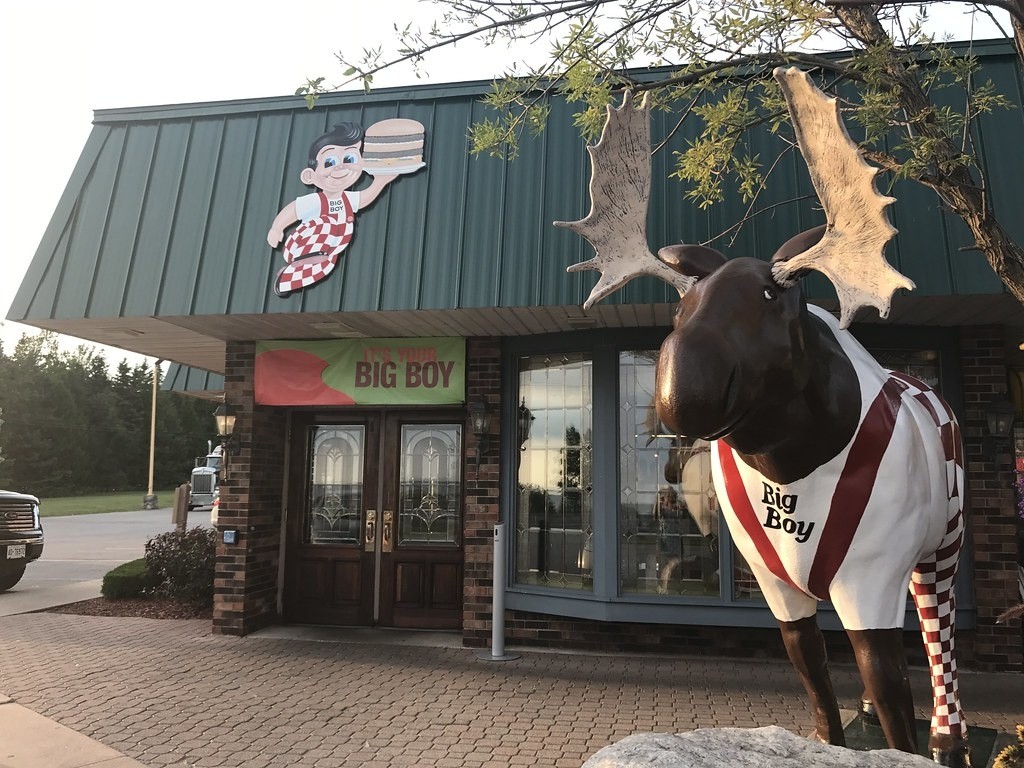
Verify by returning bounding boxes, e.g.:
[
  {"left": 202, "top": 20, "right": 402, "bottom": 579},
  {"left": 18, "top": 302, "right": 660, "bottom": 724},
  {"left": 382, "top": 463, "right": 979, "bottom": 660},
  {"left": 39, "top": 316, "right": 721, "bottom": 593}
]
[{"left": 1, "top": 490, "right": 43, "bottom": 591}]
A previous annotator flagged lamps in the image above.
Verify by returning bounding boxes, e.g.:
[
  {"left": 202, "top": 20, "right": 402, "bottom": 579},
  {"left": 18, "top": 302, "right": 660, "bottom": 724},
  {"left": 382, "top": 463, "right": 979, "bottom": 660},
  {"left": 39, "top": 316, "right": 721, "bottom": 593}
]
[
  {"left": 212, "top": 391, "right": 242, "bottom": 484},
  {"left": 465, "top": 387, "right": 493, "bottom": 493},
  {"left": 983, "top": 380, "right": 1018, "bottom": 485}
]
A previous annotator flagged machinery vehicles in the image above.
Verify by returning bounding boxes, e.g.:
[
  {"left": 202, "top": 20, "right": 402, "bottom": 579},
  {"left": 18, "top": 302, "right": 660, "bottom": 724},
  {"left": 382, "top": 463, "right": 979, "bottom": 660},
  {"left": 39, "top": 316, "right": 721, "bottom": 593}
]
[{"left": 188, "top": 441, "right": 222, "bottom": 510}]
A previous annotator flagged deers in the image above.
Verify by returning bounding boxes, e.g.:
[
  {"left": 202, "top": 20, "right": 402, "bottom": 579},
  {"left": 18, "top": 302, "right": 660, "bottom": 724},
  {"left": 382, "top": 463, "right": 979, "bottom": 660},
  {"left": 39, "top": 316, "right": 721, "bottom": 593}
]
[{"left": 551, "top": 63, "right": 971, "bottom": 768}]
